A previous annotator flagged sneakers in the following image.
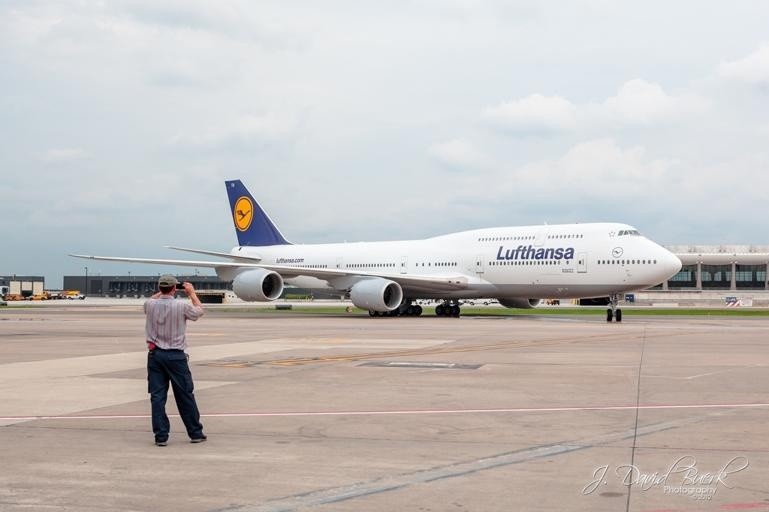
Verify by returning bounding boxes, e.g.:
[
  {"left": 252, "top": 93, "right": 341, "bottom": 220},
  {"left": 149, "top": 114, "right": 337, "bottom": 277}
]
[
  {"left": 155, "top": 436, "right": 169, "bottom": 446},
  {"left": 189, "top": 431, "right": 208, "bottom": 444}
]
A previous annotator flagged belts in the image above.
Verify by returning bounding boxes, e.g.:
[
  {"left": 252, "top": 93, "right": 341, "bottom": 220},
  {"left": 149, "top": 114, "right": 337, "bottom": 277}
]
[{"left": 152, "top": 346, "right": 184, "bottom": 353}]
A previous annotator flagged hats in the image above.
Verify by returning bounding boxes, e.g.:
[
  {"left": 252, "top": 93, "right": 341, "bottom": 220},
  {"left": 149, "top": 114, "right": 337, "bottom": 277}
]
[{"left": 157, "top": 274, "right": 183, "bottom": 289}]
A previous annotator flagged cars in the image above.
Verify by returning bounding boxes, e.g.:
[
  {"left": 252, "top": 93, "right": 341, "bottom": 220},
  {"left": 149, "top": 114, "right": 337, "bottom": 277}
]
[
  {"left": 414, "top": 298, "right": 498, "bottom": 310},
  {"left": 0, "top": 290, "right": 84, "bottom": 303}
]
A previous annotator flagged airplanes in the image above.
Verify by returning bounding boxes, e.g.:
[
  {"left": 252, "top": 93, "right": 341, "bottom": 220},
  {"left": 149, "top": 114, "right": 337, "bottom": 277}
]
[{"left": 68, "top": 179, "right": 684, "bottom": 322}]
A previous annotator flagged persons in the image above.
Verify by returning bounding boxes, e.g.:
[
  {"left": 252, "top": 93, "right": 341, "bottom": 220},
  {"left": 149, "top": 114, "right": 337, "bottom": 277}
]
[{"left": 143, "top": 274, "right": 208, "bottom": 447}]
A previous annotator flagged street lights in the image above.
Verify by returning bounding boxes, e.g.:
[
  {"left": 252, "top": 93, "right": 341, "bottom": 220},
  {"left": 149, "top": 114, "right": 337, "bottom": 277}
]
[{"left": 80, "top": 262, "right": 182, "bottom": 300}]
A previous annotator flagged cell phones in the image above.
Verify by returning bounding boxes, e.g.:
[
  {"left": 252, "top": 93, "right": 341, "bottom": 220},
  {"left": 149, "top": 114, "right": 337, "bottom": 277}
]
[{"left": 176, "top": 284, "right": 185, "bottom": 289}]
